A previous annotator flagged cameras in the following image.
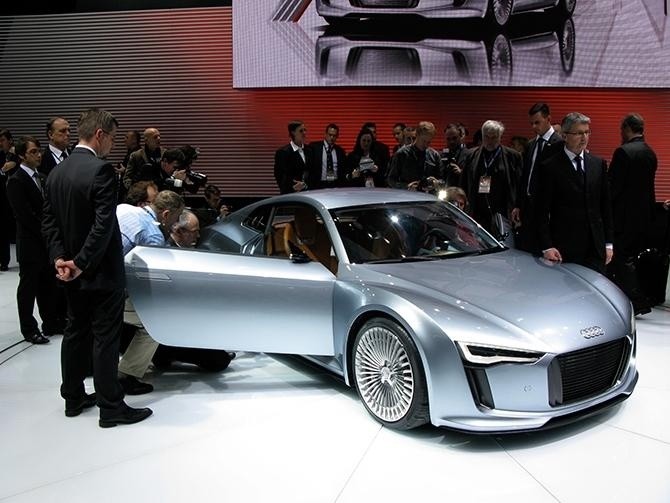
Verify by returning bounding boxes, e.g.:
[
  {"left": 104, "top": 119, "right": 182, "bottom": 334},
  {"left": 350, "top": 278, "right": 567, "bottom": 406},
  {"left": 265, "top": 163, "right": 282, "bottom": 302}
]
[
  {"left": 440, "top": 148, "right": 457, "bottom": 166},
  {"left": 183, "top": 167, "right": 207, "bottom": 186},
  {"left": 215, "top": 199, "right": 234, "bottom": 212}
]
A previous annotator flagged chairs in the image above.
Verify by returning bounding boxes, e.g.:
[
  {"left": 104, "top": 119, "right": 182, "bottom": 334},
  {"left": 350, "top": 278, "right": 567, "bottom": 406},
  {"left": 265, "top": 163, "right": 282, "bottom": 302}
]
[
  {"left": 283, "top": 207, "right": 337, "bottom": 275},
  {"left": 359, "top": 214, "right": 411, "bottom": 261}
]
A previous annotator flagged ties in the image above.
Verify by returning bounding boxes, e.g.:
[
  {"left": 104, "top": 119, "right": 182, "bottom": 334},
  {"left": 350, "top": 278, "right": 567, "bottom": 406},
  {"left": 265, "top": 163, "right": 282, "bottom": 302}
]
[
  {"left": 60, "top": 151, "right": 67, "bottom": 160},
  {"left": 34, "top": 172, "right": 41, "bottom": 191},
  {"left": 574, "top": 156, "right": 585, "bottom": 181},
  {"left": 529, "top": 138, "right": 545, "bottom": 196},
  {"left": 327, "top": 146, "right": 334, "bottom": 176}
]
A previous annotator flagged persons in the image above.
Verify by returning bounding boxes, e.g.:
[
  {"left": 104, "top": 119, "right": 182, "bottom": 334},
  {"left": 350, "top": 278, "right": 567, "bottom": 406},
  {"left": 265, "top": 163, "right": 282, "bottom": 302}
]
[
  {"left": 40, "top": 110, "right": 153, "bottom": 427},
  {"left": 151, "top": 208, "right": 231, "bottom": 371},
  {"left": 36, "top": 117, "right": 71, "bottom": 175},
  {"left": 1, "top": 128, "right": 21, "bottom": 271},
  {"left": 113, "top": 128, "right": 232, "bottom": 247},
  {"left": 606, "top": 114, "right": 658, "bottom": 315},
  {"left": 344, "top": 102, "right": 562, "bottom": 259},
  {"left": 274, "top": 120, "right": 311, "bottom": 195},
  {"left": 532, "top": 111, "right": 614, "bottom": 275},
  {"left": 307, "top": 124, "right": 345, "bottom": 190},
  {"left": 5, "top": 135, "right": 66, "bottom": 345},
  {"left": 115, "top": 190, "right": 185, "bottom": 395}
]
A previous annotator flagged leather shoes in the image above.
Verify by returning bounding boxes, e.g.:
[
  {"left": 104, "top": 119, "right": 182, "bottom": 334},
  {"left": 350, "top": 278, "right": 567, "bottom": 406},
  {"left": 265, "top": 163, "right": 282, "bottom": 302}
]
[{"left": 25, "top": 327, "right": 172, "bottom": 428}]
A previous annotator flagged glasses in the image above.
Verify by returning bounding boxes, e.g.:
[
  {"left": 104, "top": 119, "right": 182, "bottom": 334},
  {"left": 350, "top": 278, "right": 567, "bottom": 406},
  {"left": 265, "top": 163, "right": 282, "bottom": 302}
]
[
  {"left": 26, "top": 150, "right": 42, "bottom": 156},
  {"left": 567, "top": 129, "right": 592, "bottom": 137}
]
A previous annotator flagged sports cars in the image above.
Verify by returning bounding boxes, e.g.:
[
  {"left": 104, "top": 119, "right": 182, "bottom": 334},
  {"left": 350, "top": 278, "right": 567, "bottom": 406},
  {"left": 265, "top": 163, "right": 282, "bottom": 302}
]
[
  {"left": 308, "top": 0, "right": 590, "bottom": 29},
  {"left": 312, "top": 18, "right": 580, "bottom": 84},
  {"left": 123, "top": 188, "right": 640, "bottom": 439}
]
